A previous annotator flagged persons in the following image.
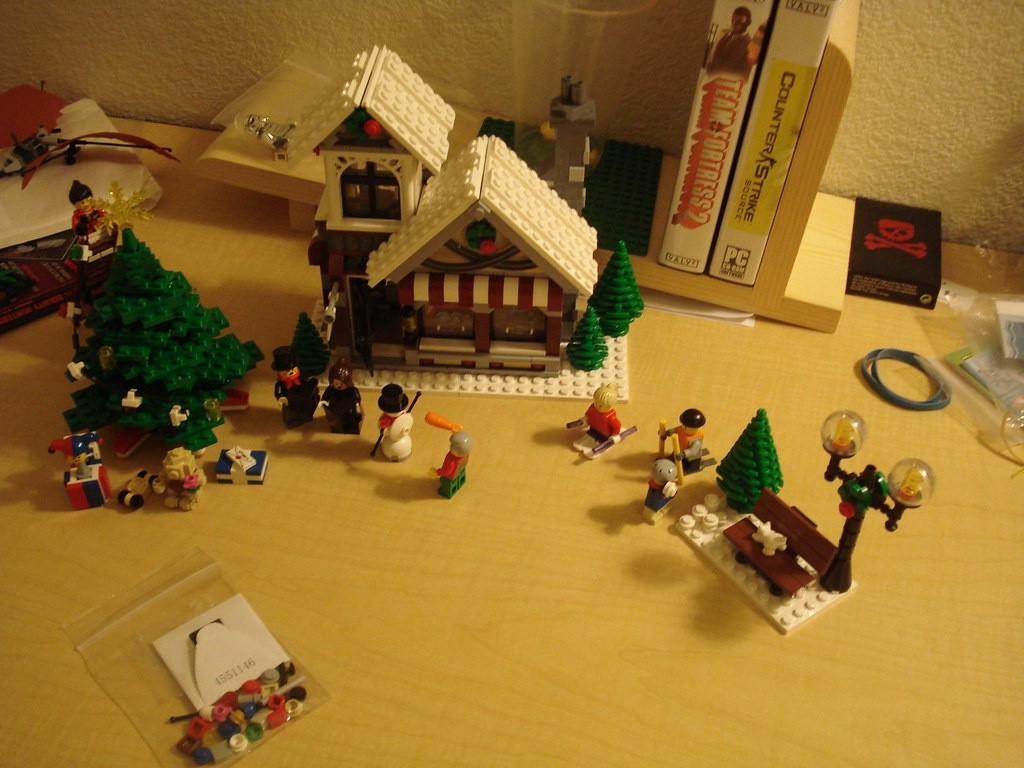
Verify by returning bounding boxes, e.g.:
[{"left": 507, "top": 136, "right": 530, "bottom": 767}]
[
  {"left": 69, "top": 179, "right": 109, "bottom": 235},
  {"left": 378, "top": 384, "right": 409, "bottom": 434},
  {"left": 650, "top": 458, "right": 679, "bottom": 511},
  {"left": 657, "top": 408, "right": 706, "bottom": 465},
  {"left": 579, "top": 385, "right": 622, "bottom": 444},
  {"left": 271, "top": 345, "right": 361, "bottom": 415},
  {"left": 433, "top": 431, "right": 471, "bottom": 480},
  {"left": 706, "top": 6, "right": 754, "bottom": 82}
]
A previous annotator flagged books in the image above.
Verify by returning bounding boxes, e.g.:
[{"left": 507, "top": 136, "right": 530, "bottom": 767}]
[{"left": 660, "top": 0, "right": 836, "bottom": 287}]
